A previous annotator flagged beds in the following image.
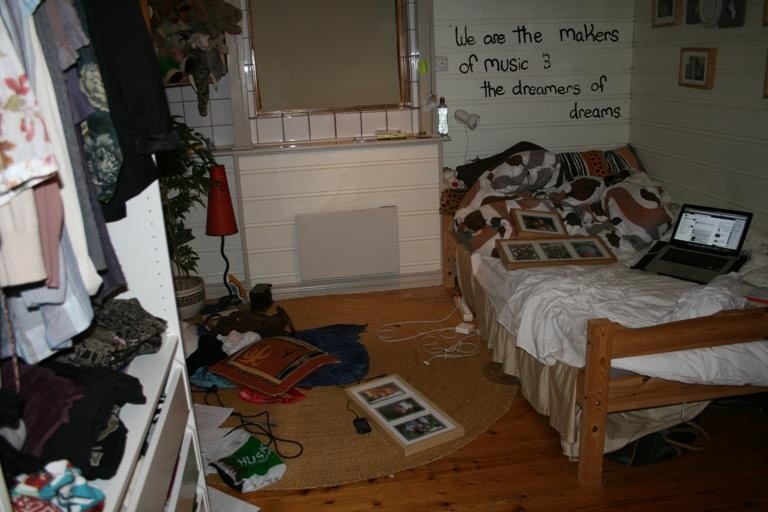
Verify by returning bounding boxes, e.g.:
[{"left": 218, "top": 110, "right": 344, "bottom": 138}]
[{"left": 442, "top": 189, "right": 768, "bottom": 490}]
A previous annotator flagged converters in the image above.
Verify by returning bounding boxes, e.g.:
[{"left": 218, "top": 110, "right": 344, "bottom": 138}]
[{"left": 456, "top": 323, "right": 474, "bottom": 335}]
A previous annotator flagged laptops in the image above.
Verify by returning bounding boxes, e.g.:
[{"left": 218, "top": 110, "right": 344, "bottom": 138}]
[{"left": 645, "top": 203, "right": 753, "bottom": 284}]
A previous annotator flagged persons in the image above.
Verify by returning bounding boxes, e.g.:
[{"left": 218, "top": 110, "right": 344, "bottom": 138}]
[
  {"left": 363, "top": 382, "right": 433, "bottom": 435},
  {"left": 525, "top": 218, "right": 539, "bottom": 229},
  {"left": 535, "top": 219, "right": 554, "bottom": 231}
]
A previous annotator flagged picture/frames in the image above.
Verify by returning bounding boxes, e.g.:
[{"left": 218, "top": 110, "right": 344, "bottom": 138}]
[
  {"left": 509, "top": 208, "right": 569, "bottom": 238},
  {"left": 495, "top": 236, "right": 617, "bottom": 271},
  {"left": 652, "top": 0, "right": 768, "bottom": 98},
  {"left": 343, "top": 375, "right": 465, "bottom": 457}
]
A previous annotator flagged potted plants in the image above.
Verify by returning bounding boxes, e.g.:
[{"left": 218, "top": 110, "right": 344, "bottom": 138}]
[{"left": 131, "top": 114, "right": 223, "bottom": 324}]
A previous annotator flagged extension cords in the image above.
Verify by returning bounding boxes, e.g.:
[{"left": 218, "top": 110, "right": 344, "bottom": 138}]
[{"left": 454, "top": 296, "right": 473, "bottom": 321}]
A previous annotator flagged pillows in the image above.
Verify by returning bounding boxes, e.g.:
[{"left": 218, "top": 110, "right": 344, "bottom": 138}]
[
  {"left": 557, "top": 144, "right": 648, "bottom": 187},
  {"left": 457, "top": 141, "right": 547, "bottom": 190}
]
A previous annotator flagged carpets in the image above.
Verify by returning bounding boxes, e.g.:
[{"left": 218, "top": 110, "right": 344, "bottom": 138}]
[{"left": 184, "top": 284, "right": 519, "bottom": 490}]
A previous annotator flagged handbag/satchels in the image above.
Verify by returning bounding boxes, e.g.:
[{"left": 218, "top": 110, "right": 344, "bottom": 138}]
[{"left": 610, "top": 419, "right": 699, "bottom": 468}]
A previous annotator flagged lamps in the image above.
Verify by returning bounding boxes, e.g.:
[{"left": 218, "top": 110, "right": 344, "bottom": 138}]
[
  {"left": 200, "top": 149, "right": 238, "bottom": 305},
  {"left": 455, "top": 109, "right": 480, "bottom": 166}
]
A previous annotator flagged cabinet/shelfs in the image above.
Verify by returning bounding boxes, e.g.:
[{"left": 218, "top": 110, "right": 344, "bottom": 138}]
[{"left": 0, "top": 154, "right": 213, "bottom": 512}]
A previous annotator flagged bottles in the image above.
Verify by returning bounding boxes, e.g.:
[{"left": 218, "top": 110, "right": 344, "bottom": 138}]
[{"left": 436, "top": 97, "right": 448, "bottom": 138}]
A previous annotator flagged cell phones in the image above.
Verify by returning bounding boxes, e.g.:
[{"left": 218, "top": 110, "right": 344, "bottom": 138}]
[{"left": 353, "top": 417, "right": 371, "bottom": 433}]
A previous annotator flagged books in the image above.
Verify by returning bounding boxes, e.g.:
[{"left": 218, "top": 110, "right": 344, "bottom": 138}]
[{"left": 376, "top": 128, "right": 406, "bottom": 141}]
